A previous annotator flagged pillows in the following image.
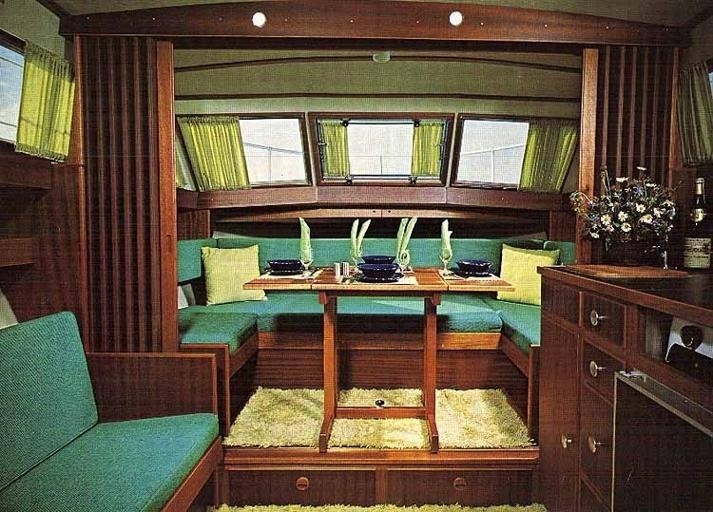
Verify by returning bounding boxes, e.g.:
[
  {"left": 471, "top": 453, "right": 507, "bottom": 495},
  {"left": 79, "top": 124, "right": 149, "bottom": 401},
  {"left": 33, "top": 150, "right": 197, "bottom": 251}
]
[
  {"left": 499, "top": 242, "right": 558, "bottom": 305},
  {"left": 202, "top": 245, "right": 267, "bottom": 304}
]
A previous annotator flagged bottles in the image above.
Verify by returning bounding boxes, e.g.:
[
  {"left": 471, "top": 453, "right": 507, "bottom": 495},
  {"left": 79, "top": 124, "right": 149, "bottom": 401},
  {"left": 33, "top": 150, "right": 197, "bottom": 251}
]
[{"left": 683, "top": 177, "right": 711, "bottom": 269}]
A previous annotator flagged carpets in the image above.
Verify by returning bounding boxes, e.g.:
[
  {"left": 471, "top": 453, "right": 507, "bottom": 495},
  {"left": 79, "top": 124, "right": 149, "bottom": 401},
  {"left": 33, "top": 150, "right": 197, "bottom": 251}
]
[
  {"left": 223, "top": 385, "right": 532, "bottom": 450},
  {"left": 205, "top": 503, "right": 548, "bottom": 512}
]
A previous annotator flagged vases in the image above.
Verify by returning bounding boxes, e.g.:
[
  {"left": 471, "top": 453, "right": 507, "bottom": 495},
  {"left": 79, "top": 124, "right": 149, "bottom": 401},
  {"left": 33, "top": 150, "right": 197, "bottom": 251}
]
[{"left": 606, "top": 232, "right": 666, "bottom": 266}]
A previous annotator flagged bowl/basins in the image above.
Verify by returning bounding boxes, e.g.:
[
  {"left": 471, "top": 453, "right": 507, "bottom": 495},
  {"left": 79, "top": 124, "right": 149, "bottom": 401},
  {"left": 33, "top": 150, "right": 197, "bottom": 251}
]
[
  {"left": 359, "top": 255, "right": 399, "bottom": 276},
  {"left": 456, "top": 260, "right": 492, "bottom": 271},
  {"left": 267, "top": 259, "right": 303, "bottom": 271}
]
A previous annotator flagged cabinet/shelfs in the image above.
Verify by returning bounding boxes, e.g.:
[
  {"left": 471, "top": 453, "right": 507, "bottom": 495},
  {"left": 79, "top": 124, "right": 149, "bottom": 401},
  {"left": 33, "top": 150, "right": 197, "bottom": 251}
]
[{"left": 536, "top": 264, "right": 713, "bottom": 512}]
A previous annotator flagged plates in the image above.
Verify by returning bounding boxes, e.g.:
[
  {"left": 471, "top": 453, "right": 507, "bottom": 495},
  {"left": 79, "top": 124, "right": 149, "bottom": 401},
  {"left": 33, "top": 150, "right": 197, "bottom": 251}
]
[
  {"left": 449, "top": 268, "right": 494, "bottom": 276},
  {"left": 264, "top": 268, "right": 302, "bottom": 275},
  {"left": 354, "top": 274, "right": 405, "bottom": 282}
]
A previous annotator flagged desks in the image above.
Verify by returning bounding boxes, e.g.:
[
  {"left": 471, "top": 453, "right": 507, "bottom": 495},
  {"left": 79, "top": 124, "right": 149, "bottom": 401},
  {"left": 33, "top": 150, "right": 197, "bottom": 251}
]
[{"left": 242, "top": 265, "right": 514, "bottom": 452}]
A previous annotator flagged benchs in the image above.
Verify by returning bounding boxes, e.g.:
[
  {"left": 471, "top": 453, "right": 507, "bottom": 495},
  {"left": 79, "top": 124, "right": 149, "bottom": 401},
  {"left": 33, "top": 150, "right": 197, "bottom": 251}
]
[
  {"left": 1, "top": 312, "right": 224, "bottom": 512},
  {"left": 170, "top": 237, "right": 574, "bottom": 440}
]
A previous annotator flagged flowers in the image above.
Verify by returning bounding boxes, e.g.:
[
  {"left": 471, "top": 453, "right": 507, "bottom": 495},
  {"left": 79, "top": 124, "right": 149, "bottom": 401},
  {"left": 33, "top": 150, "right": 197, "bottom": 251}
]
[{"left": 574, "top": 163, "right": 676, "bottom": 235}]
